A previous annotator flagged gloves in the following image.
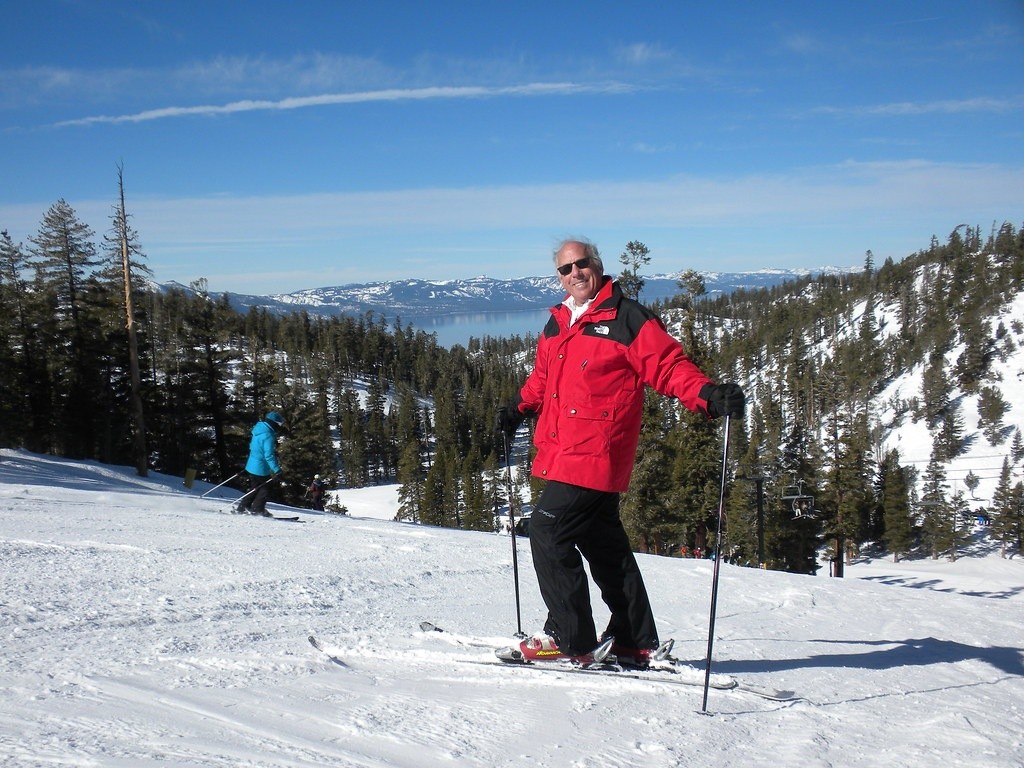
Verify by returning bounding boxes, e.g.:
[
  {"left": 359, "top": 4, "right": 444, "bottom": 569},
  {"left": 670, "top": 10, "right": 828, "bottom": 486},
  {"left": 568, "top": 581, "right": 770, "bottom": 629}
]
[
  {"left": 238, "top": 470, "right": 248, "bottom": 476},
  {"left": 275, "top": 472, "right": 285, "bottom": 480},
  {"left": 710, "top": 383, "right": 746, "bottom": 418},
  {"left": 492, "top": 406, "right": 523, "bottom": 438}
]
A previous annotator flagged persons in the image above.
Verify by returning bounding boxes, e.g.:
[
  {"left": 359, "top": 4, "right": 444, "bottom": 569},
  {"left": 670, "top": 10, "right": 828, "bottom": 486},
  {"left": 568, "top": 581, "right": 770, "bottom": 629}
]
[
  {"left": 681, "top": 545, "right": 687, "bottom": 558},
  {"left": 693, "top": 547, "right": 701, "bottom": 559},
  {"left": 722, "top": 553, "right": 729, "bottom": 563},
  {"left": 494, "top": 241, "right": 747, "bottom": 664},
  {"left": 745, "top": 560, "right": 750, "bottom": 567},
  {"left": 235, "top": 412, "right": 284, "bottom": 517},
  {"left": 759, "top": 561, "right": 766, "bottom": 570},
  {"left": 710, "top": 551, "right": 715, "bottom": 561},
  {"left": 306, "top": 474, "right": 324, "bottom": 511}
]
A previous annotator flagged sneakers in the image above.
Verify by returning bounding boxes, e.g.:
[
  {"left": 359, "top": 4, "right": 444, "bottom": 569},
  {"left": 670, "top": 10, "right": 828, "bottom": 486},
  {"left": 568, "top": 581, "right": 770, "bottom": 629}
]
[
  {"left": 519, "top": 630, "right": 596, "bottom": 661},
  {"left": 601, "top": 638, "right": 676, "bottom": 669}
]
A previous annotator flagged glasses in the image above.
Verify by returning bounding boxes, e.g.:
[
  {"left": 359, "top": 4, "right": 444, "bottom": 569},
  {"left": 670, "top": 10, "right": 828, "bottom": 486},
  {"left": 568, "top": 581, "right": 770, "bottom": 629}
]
[{"left": 557, "top": 258, "right": 592, "bottom": 275}]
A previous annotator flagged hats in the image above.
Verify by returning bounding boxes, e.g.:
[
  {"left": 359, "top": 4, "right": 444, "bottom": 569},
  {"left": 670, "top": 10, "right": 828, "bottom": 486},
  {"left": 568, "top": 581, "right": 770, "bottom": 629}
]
[
  {"left": 267, "top": 410, "right": 287, "bottom": 424},
  {"left": 313, "top": 474, "right": 323, "bottom": 479}
]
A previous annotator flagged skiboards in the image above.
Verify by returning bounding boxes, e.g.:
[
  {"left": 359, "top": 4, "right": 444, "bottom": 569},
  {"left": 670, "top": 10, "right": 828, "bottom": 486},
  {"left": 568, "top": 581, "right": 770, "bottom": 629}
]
[
  {"left": 200, "top": 505, "right": 300, "bottom": 523},
  {"left": 419, "top": 619, "right": 803, "bottom": 702}
]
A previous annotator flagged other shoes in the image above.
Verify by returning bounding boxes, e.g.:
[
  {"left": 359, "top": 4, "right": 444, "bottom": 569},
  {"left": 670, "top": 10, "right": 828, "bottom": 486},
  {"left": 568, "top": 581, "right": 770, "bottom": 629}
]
[
  {"left": 253, "top": 510, "right": 272, "bottom": 518},
  {"left": 238, "top": 505, "right": 254, "bottom": 514}
]
